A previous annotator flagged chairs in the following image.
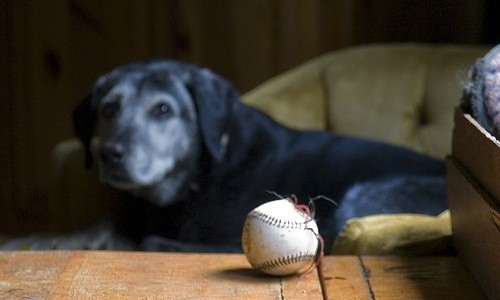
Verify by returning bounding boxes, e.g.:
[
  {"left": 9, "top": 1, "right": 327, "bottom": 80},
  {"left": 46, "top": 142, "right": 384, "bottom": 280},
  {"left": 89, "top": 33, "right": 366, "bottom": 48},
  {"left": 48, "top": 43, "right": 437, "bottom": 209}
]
[{"left": 241, "top": 37, "right": 490, "bottom": 163}]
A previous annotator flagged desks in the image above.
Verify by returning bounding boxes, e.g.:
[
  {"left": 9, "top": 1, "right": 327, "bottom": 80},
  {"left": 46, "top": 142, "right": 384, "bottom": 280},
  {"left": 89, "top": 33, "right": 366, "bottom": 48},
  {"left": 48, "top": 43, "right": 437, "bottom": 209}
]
[{"left": 1, "top": 250, "right": 487, "bottom": 299}]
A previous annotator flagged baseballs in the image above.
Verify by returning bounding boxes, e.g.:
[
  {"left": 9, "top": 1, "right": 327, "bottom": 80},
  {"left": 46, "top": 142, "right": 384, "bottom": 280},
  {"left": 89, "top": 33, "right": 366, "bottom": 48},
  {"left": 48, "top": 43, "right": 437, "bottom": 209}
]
[{"left": 241, "top": 196, "right": 319, "bottom": 279}]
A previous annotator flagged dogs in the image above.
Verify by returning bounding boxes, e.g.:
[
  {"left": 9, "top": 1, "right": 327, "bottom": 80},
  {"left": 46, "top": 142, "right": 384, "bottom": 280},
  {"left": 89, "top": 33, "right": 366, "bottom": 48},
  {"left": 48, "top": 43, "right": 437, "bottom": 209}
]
[{"left": 71, "top": 60, "right": 451, "bottom": 252}]
